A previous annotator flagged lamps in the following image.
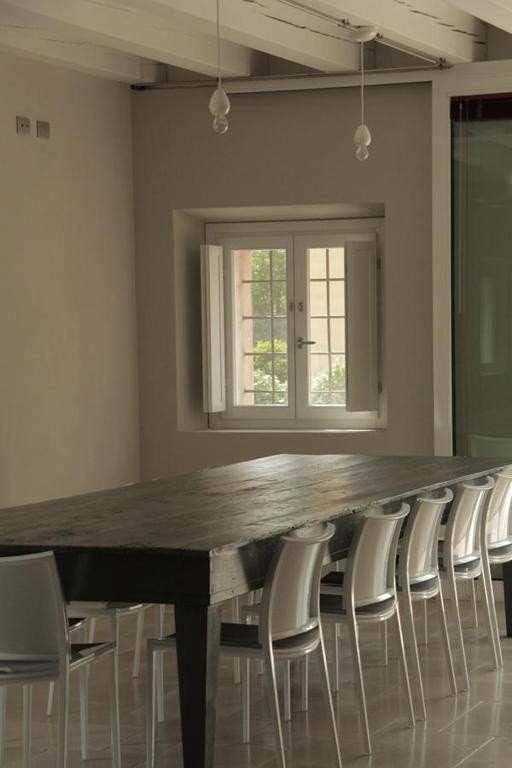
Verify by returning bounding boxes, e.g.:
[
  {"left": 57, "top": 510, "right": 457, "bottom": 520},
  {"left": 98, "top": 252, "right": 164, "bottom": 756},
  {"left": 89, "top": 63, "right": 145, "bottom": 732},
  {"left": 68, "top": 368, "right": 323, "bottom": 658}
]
[
  {"left": 208, "top": 0, "right": 231, "bottom": 135},
  {"left": 351, "top": 30, "right": 374, "bottom": 161}
]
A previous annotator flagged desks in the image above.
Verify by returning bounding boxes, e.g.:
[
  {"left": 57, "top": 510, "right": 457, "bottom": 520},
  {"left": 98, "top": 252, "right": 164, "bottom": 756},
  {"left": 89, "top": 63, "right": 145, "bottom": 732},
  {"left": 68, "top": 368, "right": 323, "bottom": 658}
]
[{"left": 5, "top": 452, "right": 506, "bottom": 768}]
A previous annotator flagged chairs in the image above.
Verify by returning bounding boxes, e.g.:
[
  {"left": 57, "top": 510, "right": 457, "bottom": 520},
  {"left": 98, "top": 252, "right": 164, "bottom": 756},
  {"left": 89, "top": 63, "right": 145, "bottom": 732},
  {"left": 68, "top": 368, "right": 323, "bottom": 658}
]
[
  {"left": 39, "top": 595, "right": 157, "bottom": 715},
  {"left": 242, "top": 503, "right": 415, "bottom": 743},
  {"left": 375, "top": 473, "right": 500, "bottom": 674},
  {"left": 399, "top": 469, "right": 511, "bottom": 648},
  {"left": 300, "top": 485, "right": 458, "bottom": 713},
  {"left": 147, "top": 520, "right": 343, "bottom": 768},
  {"left": 0, "top": 550, "right": 126, "bottom": 768}
]
